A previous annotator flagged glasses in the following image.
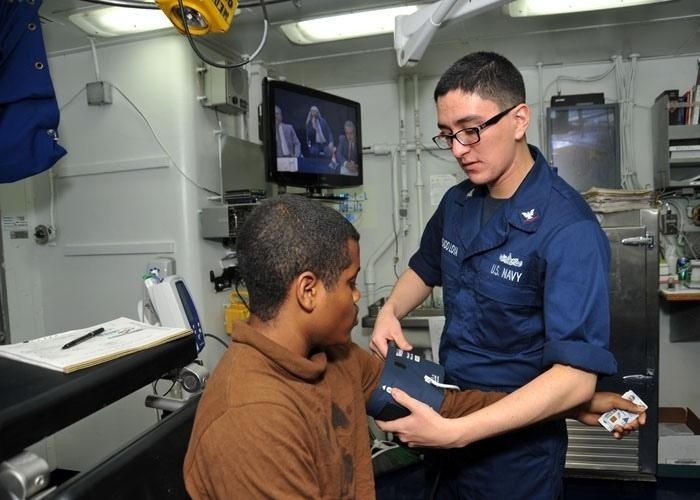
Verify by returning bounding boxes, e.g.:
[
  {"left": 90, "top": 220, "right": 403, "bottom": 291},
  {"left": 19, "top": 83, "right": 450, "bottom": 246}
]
[{"left": 432, "top": 107, "right": 514, "bottom": 149}]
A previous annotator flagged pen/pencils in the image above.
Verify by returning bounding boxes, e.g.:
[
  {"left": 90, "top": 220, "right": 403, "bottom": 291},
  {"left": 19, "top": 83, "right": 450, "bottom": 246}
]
[{"left": 62, "top": 327, "right": 104, "bottom": 350}]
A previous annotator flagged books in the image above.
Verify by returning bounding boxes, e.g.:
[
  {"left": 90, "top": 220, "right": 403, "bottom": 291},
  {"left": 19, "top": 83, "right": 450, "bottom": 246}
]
[{"left": 655, "top": 85, "right": 699, "bottom": 159}]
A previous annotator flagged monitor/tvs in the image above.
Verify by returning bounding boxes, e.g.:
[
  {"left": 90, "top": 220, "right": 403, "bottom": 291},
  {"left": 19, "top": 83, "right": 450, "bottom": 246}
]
[{"left": 261, "top": 76, "right": 364, "bottom": 199}]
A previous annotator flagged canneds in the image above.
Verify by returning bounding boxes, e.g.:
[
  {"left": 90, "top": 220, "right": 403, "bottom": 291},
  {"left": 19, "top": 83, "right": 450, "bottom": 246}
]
[{"left": 677, "top": 256, "right": 690, "bottom": 280}]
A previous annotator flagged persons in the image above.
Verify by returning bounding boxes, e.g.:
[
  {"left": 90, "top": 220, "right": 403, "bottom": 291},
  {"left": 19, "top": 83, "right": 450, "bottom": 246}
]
[
  {"left": 275, "top": 105, "right": 301, "bottom": 159},
  {"left": 368, "top": 51, "right": 618, "bottom": 500},
  {"left": 334, "top": 119, "right": 359, "bottom": 171},
  {"left": 183, "top": 195, "right": 647, "bottom": 500},
  {"left": 305, "top": 105, "right": 335, "bottom": 159}
]
